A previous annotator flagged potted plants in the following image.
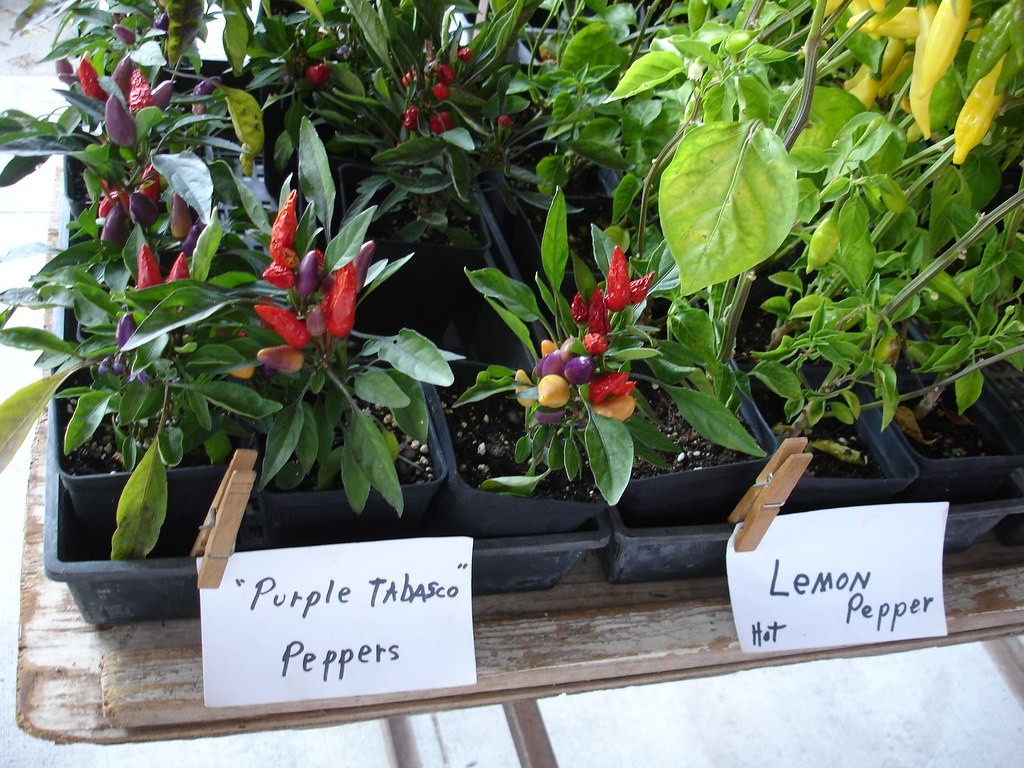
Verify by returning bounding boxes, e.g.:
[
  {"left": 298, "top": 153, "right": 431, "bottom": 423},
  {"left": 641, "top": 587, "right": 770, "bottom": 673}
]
[{"left": 2, "top": 0, "right": 1024, "bottom": 630}]
